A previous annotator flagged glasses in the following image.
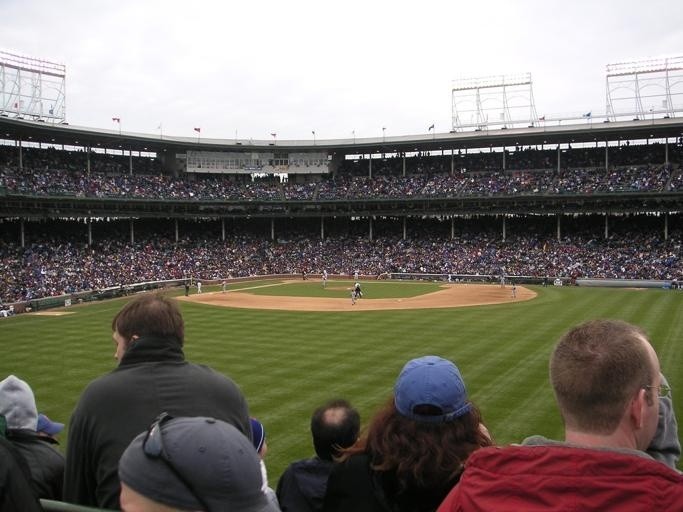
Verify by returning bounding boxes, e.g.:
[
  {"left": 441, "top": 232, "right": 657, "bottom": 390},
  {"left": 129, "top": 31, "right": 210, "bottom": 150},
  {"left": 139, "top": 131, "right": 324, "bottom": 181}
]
[
  {"left": 632, "top": 385, "right": 671, "bottom": 405},
  {"left": 143, "top": 412, "right": 210, "bottom": 511}
]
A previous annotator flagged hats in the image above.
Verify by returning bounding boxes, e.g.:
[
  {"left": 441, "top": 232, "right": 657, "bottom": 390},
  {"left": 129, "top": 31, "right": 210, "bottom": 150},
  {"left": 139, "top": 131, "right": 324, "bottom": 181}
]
[
  {"left": 118, "top": 416, "right": 280, "bottom": 512},
  {"left": 394, "top": 355, "right": 472, "bottom": 423},
  {"left": 37, "top": 414, "right": 65, "bottom": 434}
]
